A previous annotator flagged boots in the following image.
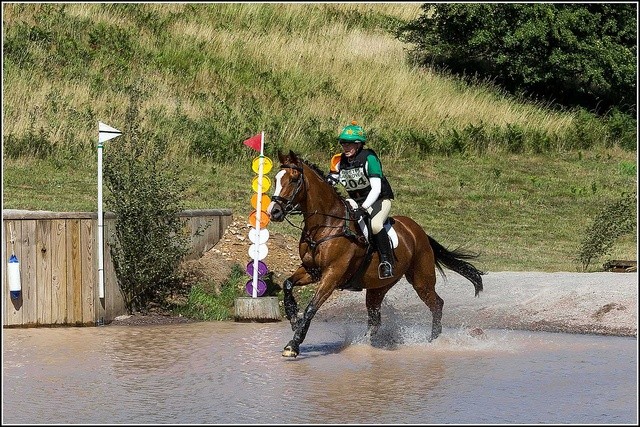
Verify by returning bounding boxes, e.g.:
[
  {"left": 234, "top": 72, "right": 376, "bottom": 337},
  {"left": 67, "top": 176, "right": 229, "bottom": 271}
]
[{"left": 373, "top": 228, "right": 396, "bottom": 275}]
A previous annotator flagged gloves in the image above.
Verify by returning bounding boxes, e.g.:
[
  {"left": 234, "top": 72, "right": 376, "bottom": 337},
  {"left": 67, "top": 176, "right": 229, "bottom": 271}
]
[{"left": 352, "top": 207, "right": 369, "bottom": 219}]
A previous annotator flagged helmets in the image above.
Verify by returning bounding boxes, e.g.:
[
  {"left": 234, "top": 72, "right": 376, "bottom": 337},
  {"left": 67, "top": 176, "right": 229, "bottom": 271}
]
[{"left": 337, "top": 124, "right": 367, "bottom": 143}]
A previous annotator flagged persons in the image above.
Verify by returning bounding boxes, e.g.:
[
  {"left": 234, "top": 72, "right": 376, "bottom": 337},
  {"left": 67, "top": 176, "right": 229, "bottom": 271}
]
[{"left": 327, "top": 120, "right": 396, "bottom": 278}]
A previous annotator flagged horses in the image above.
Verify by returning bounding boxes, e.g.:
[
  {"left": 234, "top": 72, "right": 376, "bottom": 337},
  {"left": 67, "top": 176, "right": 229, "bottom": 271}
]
[{"left": 266, "top": 150, "right": 484, "bottom": 358}]
